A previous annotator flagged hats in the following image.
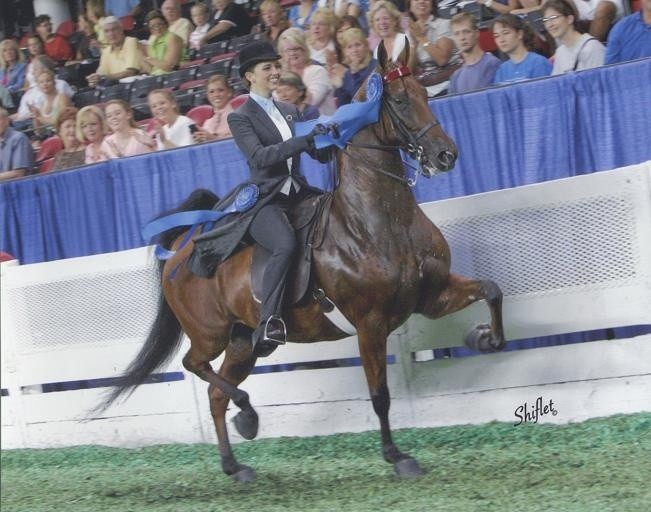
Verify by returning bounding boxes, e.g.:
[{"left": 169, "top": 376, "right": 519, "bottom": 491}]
[{"left": 238, "top": 42, "right": 281, "bottom": 77}]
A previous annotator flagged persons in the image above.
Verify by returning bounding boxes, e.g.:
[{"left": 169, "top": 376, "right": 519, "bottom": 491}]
[
  {"left": 228, "top": 43, "right": 338, "bottom": 358},
  {"left": 0, "top": 0, "right": 651, "bottom": 181}
]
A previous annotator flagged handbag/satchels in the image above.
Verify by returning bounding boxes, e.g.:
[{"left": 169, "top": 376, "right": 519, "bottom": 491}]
[{"left": 415, "top": 49, "right": 464, "bottom": 86}]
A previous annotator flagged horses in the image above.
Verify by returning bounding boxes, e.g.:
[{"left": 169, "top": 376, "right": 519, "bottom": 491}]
[{"left": 87, "top": 35, "right": 506, "bottom": 486}]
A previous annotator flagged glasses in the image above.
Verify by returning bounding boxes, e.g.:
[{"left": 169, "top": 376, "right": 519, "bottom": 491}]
[{"left": 542, "top": 15, "right": 562, "bottom": 23}]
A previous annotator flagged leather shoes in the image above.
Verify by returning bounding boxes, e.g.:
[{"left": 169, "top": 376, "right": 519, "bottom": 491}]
[{"left": 259, "top": 329, "right": 285, "bottom": 349}]
